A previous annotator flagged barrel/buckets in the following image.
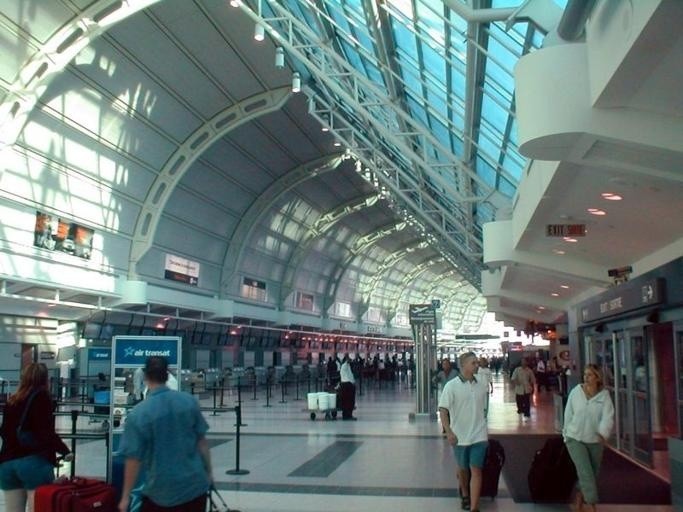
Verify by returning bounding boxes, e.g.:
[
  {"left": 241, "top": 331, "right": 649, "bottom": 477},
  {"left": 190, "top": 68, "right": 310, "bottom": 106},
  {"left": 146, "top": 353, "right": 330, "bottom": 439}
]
[{"left": 307, "top": 391, "right": 337, "bottom": 411}]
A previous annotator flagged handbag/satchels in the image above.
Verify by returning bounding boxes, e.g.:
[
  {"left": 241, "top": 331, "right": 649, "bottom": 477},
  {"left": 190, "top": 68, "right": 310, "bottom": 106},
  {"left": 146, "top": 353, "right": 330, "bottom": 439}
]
[{"left": 18, "top": 425, "right": 34, "bottom": 443}]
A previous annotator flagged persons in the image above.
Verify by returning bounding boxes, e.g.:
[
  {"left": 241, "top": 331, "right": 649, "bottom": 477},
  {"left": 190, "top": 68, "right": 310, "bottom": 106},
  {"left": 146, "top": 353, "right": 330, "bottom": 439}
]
[
  {"left": 118, "top": 356, "right": 214, "bottom": 512},
  {"left": 439, "top": 352, "right": 489, "bottom": 512},
  {"left": 561, "top": 363, "right": 614, "bottom": 512},
  {"left": 327, "top": 356, "right": 560, "bottom": 433},
  {"left": 0, "top": 363, "right": 74, "bottom": 512}
]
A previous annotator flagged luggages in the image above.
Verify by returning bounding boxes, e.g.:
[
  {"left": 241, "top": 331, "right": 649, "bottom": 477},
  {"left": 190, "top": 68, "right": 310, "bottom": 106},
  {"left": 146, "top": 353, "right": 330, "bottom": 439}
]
[
  {"left": 35, "top": 455, "right": 117, "bottom": 512},
  {"left": 527, "top": 434, "right": 580, "bottom": 503},
  {"left": 460, "top": 439, "right": 504, "bottom": 497}
]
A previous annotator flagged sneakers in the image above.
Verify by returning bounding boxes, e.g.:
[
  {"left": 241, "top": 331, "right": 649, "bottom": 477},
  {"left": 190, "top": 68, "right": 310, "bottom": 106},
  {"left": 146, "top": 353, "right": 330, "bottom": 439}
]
[
  {"left": 343, "top": 415, "right": 355, "bottom": 420},
  {"left": 518, "top": 414, "right": 528, "bottom": 421}
]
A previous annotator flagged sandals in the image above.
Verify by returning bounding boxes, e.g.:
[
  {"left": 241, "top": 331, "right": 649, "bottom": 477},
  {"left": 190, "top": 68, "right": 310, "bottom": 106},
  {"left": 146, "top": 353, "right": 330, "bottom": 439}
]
[{"left": 461, "top": 497, "right": 470, "bottom": 510}]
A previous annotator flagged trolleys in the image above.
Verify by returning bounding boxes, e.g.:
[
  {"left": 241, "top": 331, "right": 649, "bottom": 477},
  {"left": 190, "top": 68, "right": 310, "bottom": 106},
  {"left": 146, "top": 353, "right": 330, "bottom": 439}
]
[{"left": 302, "top": 379, "right": 343, "bottom": 420}]
